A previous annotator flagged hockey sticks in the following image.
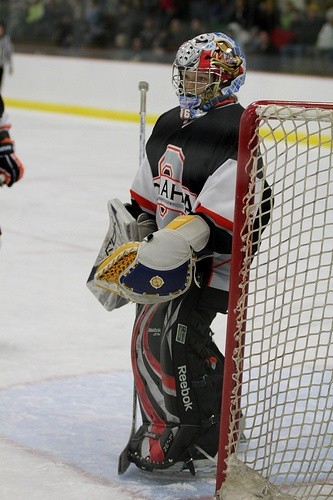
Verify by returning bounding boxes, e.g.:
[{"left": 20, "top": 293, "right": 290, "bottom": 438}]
[{"left": 117, "top": 81, "right": 149, "bottom": 476}]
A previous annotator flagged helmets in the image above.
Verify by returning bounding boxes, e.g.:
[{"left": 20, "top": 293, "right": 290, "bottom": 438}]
[{"left": 171, "top": 31, "right": 248, "bottom": 114}]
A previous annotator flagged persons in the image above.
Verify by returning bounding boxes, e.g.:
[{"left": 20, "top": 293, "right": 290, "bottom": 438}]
[
  {"left": 86, "top": 31, "right": 274, "bottom": 470},
  {"left": 0, "top": 0, "right": 333, "bottom": 188}
]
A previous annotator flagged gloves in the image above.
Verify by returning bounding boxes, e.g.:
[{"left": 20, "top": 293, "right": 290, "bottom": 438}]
[{"left": 0, "top": 123, "right": 25, "bottom": 187}]
